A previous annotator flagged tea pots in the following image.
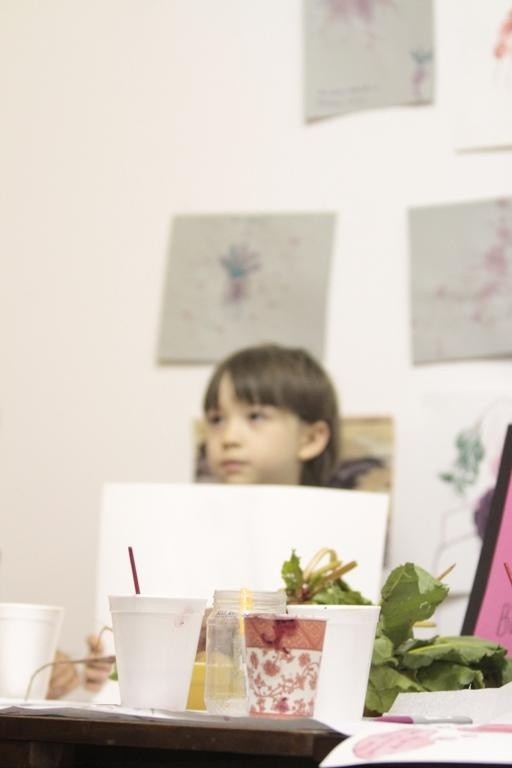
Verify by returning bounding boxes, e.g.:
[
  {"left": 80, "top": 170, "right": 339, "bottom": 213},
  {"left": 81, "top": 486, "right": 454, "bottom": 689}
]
[{"left": 244, "top": 614, "right": 327, "bottom": 720}]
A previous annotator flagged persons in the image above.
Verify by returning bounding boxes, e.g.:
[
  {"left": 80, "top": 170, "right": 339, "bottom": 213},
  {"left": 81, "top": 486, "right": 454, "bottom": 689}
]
[{"left": 84, "top": 345, "right": 340, "bottom": 692}]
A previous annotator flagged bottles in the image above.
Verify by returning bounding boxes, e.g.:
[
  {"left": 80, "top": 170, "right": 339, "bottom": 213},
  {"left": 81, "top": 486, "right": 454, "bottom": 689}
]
[
  {"left": 250, "top": 589, "right": 285, "bottom": 615},
  {"left": 203, "top": 588, "right": 252, "bottom": 715}
]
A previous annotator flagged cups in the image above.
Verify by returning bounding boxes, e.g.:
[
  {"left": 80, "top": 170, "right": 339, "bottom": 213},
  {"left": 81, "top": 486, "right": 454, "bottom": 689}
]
[
  {"left": 0, "top": 602, "right": 65, "bottom": 707},
  {"left": 108, "top": 596, "right": 209, "bottom": 712}
]
